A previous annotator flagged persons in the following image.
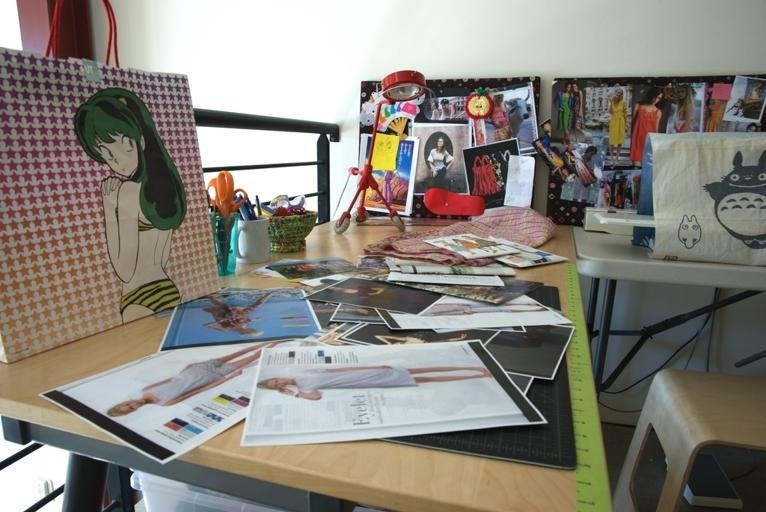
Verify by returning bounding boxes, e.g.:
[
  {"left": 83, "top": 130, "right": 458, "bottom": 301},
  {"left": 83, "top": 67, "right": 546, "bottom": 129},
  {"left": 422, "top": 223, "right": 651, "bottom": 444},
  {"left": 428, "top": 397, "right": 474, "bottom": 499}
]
[
  {"left": 536, "top": 82, "right": 696, "bottom": 210},
  {"left": 74, "top": 88, "right": 187, "bottom": 324},
  {"left": 427, "top": 136, "right": 454, "bottom": 185},
  {"left": 704, "top": 92, "right": 725, "bottom": 132},
  {"left": 257, "top": 364, "right": 492, "bottom": 401},
  {"left": 202, "top": 292, "right": 271, "bottom": 336},
  {"left": 107, "top": 340, "right": 292, "bottom": 417},
  {"left": 726, "top": 81, "right": 765, "bottom": 116},
  {"left": 439, "top": 99, "right": 456, "bottom": 120},
  {"left": 431, "top": 102, "right": 439, "bottom": 120},
  {"left": 295, "top": 236, "right": 507, "bottom": 345},
  {"left": 485, "top": 94, "right": 511, "bottom": 141},
  {"left": 746, "top": 123, "right": 758, "bottom": 132}
]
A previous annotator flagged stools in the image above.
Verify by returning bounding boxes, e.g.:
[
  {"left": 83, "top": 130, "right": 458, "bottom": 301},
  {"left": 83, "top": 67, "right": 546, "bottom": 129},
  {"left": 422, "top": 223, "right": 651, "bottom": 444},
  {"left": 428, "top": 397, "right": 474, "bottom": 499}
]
[{"left": 613, "top": 367, "right": 765, "bottom": 510}]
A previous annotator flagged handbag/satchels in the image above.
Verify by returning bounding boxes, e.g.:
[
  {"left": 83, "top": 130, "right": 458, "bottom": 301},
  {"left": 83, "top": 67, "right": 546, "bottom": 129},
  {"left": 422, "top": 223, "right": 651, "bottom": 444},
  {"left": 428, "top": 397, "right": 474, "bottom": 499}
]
[{"left": 1, "top": 0, "right": 220, "bottom": 364}]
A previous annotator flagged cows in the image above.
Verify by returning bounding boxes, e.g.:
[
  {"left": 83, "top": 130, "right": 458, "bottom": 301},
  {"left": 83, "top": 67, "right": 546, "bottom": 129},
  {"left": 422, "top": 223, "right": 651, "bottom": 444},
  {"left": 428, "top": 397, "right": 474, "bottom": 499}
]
[{"left": 503, "top": 88, "right": 531, "bottom": 138}]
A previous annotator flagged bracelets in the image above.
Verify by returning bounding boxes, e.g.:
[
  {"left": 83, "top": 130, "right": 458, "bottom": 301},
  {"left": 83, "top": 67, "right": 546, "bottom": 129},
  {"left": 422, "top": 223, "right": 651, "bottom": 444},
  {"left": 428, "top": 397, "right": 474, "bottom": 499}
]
[{"left": 294, "top": 392, "right": 299, "bottom": 398}]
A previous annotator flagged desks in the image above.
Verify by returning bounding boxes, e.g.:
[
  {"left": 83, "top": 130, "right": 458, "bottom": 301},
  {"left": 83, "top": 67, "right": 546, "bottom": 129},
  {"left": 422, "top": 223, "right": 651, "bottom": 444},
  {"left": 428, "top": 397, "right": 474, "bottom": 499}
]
[{"left": 3, "top": 220, "right": 766, "bottom": 510}]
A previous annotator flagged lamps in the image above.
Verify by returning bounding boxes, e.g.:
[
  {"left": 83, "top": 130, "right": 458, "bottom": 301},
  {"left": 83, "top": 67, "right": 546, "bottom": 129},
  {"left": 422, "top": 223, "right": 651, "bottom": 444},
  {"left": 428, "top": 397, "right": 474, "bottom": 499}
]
[{"left": 335, "top": 69, "right": 427, "bottom": 234}]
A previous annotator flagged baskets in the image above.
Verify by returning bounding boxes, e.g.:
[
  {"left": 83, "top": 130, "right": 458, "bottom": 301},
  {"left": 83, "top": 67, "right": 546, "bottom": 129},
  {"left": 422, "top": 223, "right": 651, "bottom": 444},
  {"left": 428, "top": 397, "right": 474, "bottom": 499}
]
[{"left": 260, "top": 208, "right": 318, "bottom": 253}]
